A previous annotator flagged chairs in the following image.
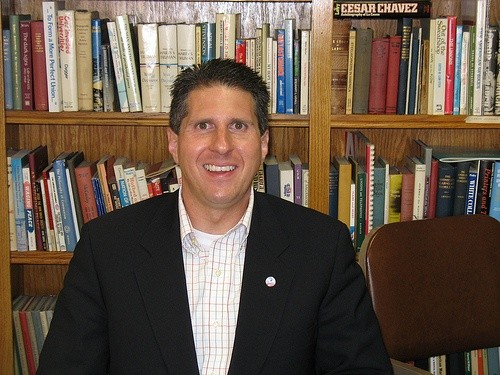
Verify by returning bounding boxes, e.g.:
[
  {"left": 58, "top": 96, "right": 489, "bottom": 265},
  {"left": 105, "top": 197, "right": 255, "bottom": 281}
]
[{"left": 359, "top": 214, "right": 500, "bottom": 374}]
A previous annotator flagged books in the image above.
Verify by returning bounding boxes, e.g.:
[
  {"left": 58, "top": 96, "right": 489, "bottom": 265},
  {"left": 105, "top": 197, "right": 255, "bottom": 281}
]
[
  {"left": 11, "top": 293, "right": 59, "bottom": 375},
  {"left": 6, "top": 130, "right": 499, "bottom": 255},
  {"left": 417, "top": 347, "right": 500, "bottom": 375},
  {"left": 0, "top": 0, "right": 500, "bottom": 117}
]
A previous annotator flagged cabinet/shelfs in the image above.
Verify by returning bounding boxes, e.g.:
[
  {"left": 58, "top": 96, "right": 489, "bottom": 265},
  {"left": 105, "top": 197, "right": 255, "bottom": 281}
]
[
  {"left": 0, "top": 0, "right": 311, "bottom": 375},
  {"left": 329, "top": 0, "right": 500, "bottom": 266}
]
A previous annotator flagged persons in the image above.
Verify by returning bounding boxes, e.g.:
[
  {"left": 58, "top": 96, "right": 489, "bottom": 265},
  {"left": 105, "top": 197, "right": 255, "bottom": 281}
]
[{"left": 36, "top": 59, "right": 394, "bottom": 374}]
